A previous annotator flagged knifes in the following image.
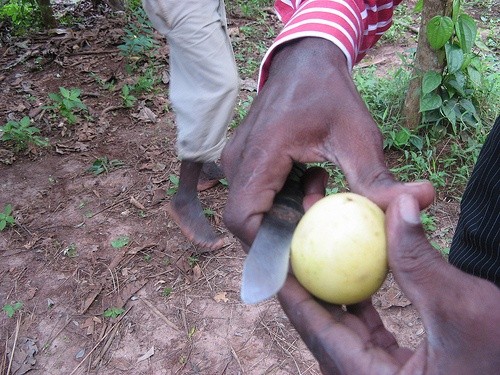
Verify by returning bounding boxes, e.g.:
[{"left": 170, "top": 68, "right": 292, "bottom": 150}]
[{"left": 240, "top": 162, "right": 309, "bottom": 304}]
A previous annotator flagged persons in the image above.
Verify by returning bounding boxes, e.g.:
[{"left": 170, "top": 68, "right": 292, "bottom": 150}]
[
  {"left": 141, "top": 0, "right": 241, "bottom": 250},
  {"left": 222, "top": 0, "right": 500, "bottom": 375}
]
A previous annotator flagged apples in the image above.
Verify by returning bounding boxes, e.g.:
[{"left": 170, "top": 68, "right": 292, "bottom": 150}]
[{"left": 290, "top": 192, "right": 390, "bottom": 306}]
